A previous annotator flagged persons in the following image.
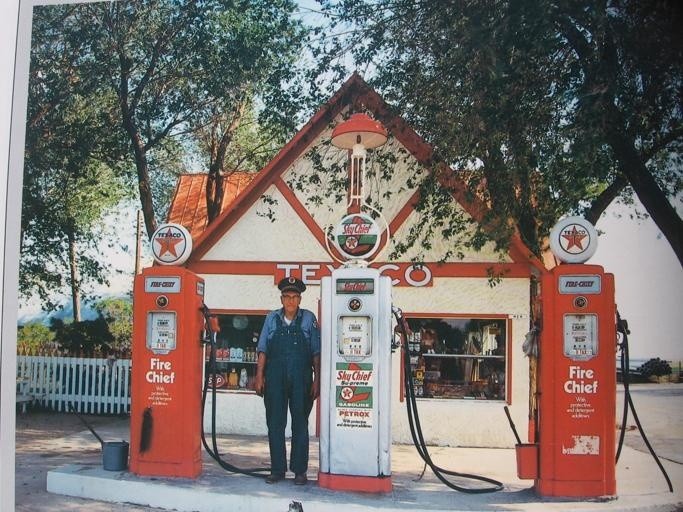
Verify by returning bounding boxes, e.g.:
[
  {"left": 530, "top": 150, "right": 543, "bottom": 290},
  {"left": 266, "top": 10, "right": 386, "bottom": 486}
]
[{"left": 253, "top": 276, "right": 320, "bottom": 485}]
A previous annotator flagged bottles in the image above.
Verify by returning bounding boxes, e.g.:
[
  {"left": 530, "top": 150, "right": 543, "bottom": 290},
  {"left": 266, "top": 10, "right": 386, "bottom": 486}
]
[{"left": 229, "top": 345, "right": 256, "bottom": 361}]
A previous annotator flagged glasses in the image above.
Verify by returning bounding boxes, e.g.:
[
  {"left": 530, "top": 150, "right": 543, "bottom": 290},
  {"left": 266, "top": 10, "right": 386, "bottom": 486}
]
[{"left": 282, "top": 294, "right": 303, "bottom": 301}]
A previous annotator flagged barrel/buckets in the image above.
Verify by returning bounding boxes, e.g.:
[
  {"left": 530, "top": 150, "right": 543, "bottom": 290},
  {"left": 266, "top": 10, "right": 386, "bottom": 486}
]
[{"left": 102, "top": 441, "right": 129, "bottom": 472}]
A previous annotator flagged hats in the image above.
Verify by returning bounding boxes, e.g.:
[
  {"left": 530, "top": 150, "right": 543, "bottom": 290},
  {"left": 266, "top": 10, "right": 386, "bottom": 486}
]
[{"left": 277, "top": 277, "right": 306, "bottom": 293}]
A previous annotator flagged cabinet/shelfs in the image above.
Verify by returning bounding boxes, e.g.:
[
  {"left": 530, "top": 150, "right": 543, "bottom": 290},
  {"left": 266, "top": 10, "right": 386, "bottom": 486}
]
[{"left": 206, "top": 359, "right": 257, "bottom": 393}]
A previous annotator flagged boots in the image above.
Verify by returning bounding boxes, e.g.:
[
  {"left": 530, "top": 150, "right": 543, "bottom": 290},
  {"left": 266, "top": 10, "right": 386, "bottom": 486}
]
[
  {"left": 265, "top": 470, "right": 286, "bottom": 484},
  {"left": 293, "top": 471, "right": 307, "bottom": 485}
]
